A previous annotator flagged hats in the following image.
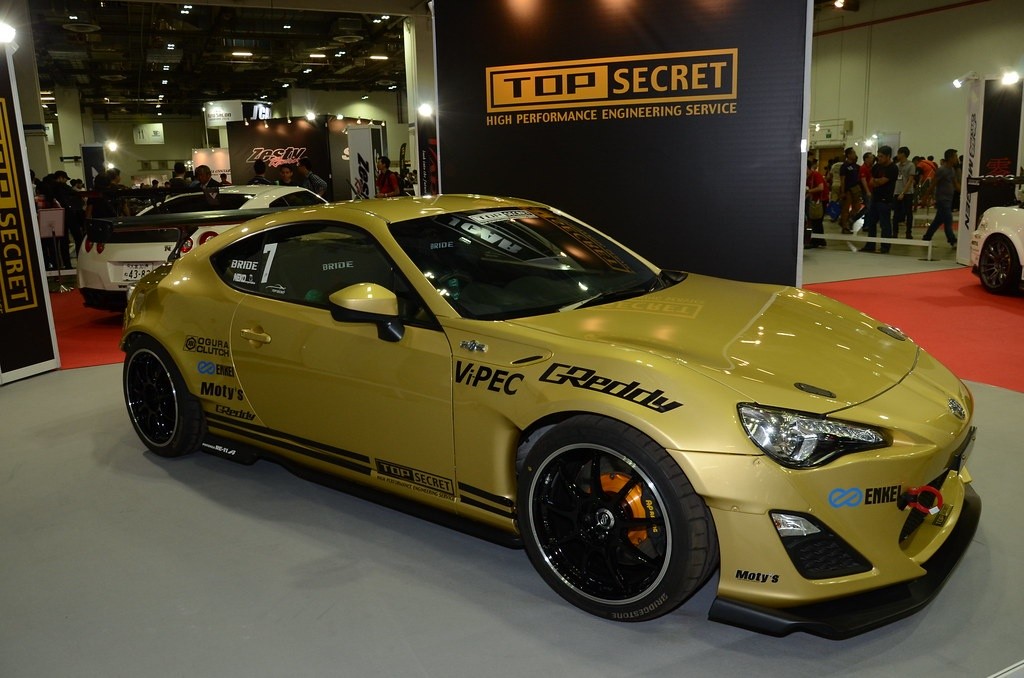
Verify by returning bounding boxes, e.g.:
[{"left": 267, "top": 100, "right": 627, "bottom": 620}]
[{"left": 55, "top": 171, "right": 71, "bottom": 180}]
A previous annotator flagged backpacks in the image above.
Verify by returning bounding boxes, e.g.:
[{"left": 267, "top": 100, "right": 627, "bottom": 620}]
[{"left": 385, "top": 171, "right": 404, "bottom": 196}]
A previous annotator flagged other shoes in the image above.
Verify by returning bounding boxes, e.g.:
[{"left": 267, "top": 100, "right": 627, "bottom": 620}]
[
  {"left": 880, "top": 246, "right": 889, "bottom": 253},
  {"left": 893, "top": 232, "right": 897, "bottom": 238},
  {"left": 906, "top": 232, "right": 912, "bottom": 239},
  {"left": 862, "top": 243, "right": 876, "bottom": 251},
  {"left": 842, "top": 228, "right": 853, "bottom": 234},
  {"left": 838, "top": 221, "right": 842, "bottom": 226},
  {"left": 811, "top": 241, "right": 827, "bottom": 247},
  {"left": 951, "top": 238, "right": 957, "bottom": 245}
]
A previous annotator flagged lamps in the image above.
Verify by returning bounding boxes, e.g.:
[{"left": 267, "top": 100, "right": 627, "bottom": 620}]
[
  {"left": 953, "top": 71, "right": 980, "bottom": 88},
  {"left": 62, "top": 0, "right": 103, "bottom": 33},
  {"left": 243, "top": 114, "right": 385, "bottom": 128},
  {"left": 226, "top": 38, "right": 254, "bottom": 58},
  {"left": 333, "top": 17, "right": 368, "bottom": 44},
  {"left": 368, "top": 42, "right": 389, "bottom": 63},
  {"left": 98, "top": 59, "right": 127, "bottom": 82}
]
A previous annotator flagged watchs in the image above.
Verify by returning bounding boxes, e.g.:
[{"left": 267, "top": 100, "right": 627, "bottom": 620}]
[{"left": 901, "top": 192, "right": 904, "bottom": 195}]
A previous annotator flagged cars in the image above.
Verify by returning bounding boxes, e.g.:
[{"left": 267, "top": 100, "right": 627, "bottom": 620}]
[
  {"left": 969, "top": 206, "right": 1024, "bottom": 297},
  {"left": 77, "top": 181, "right": 357, "bottom": 313}
]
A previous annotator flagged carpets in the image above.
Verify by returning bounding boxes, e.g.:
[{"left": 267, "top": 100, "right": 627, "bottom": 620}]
[
  {"left": 49, "top": 285, "right": 129, "bottom": 368},
  {"left": 802, "top": 264, "right": 1024, "bottom": 397}
]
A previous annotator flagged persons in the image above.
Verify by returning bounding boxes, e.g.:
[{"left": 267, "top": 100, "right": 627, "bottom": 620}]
[
  {"left": 247, "top": 159, "right": 327, "bottom": 200},
  {"left": 393, "top": 170, "right": 417, "bottom": 196},
  {"left": 219, "top": 174, "right": 232, "bottom": 186},
  {"left": 27, "top": 162, "right": 221, "bottom": 292},
  {"left": 804, "top": 145, "right": 964, "bottom": 253},
  {"left": 374, "top": 156, "right": 400, "bottom": 198}
]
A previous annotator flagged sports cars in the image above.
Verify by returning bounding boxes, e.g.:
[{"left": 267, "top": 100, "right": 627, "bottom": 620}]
[{"left": 118, "top": 194, "right": 982, "bottom": 642}]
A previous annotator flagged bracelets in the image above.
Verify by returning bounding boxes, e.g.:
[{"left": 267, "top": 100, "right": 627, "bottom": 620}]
[{"left": 873, "top": 178, "right": 876, "bottom": 184}]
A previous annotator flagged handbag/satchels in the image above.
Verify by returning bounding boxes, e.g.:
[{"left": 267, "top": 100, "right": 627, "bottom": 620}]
[
  {"left": 808, "top": 201, "right": 823, "bottom": 219},
  {"left": 825, "top": 199, "right": 842, "bottom": 221}
]
